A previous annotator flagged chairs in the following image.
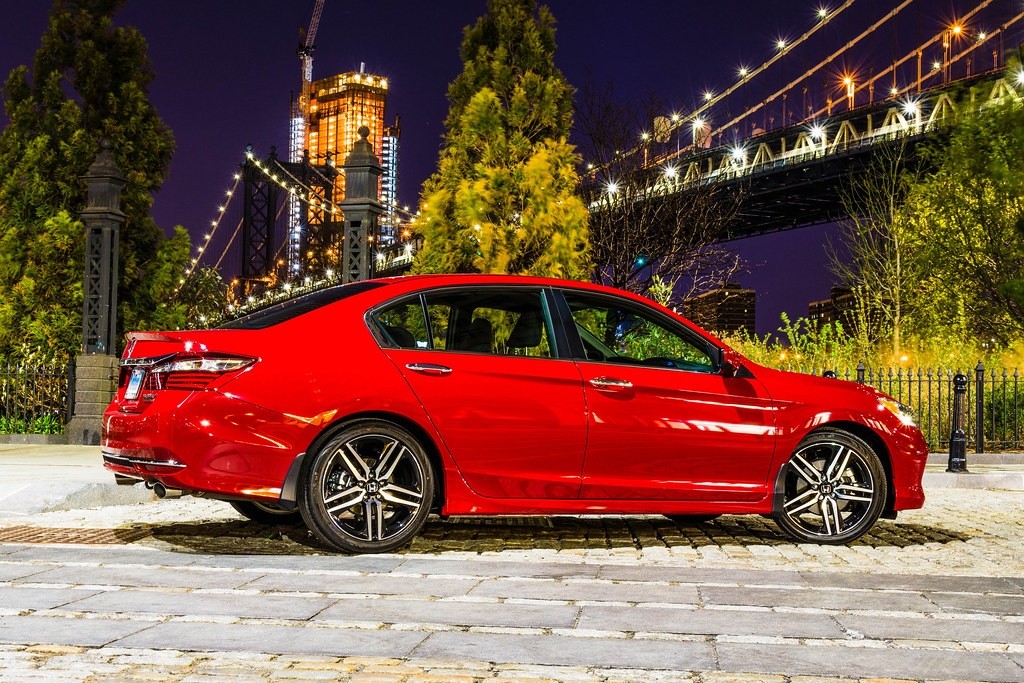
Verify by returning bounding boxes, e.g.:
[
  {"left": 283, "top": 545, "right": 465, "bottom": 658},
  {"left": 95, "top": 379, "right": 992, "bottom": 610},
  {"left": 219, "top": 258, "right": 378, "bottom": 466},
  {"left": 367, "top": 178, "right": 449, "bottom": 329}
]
[
  {"left": 505, "top": 310, "right": 545, "bottom": 356},
  {"left": 471, "top": 318, "right": 495, "bottom": 354},
  {"left": 388, "top": 326, "right": 416, "bottom": 348}
]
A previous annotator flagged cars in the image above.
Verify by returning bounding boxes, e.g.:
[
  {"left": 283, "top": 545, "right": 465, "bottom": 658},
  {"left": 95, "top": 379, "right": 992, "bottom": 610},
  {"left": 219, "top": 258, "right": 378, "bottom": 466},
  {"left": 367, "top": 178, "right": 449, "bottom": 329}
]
[{"left": 98, "top": 272, "right": 930, "bottom": 555}]
